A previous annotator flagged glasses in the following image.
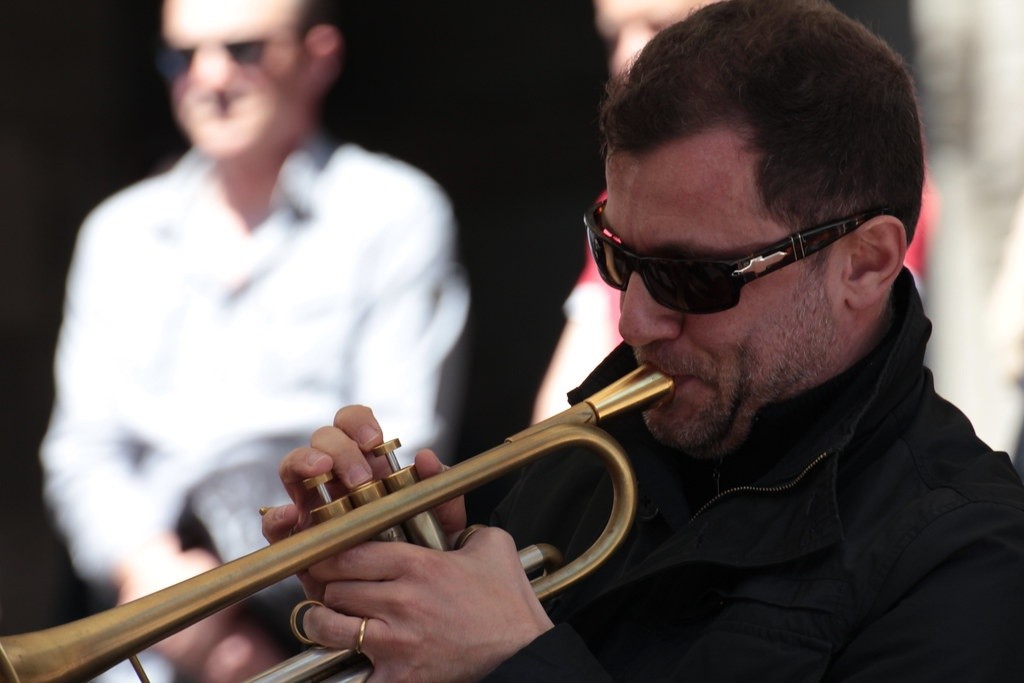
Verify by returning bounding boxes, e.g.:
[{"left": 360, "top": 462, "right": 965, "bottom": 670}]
[
  {"left": 583, "top": 198, "right": 881, "bottom": 314},
  {"left": 156, "top": 32, "right": 307, "bottom": 82}
]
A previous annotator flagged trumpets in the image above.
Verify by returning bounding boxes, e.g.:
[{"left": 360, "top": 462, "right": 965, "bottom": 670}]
[{"left": 0, "top": 361, "right": 678, "bottom": 683}]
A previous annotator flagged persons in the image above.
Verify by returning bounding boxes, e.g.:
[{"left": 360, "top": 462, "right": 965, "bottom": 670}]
[
  {"left": 530, "top": 0, "right": 928, "bottom": 427},
  {"left": 40, "top": 0, "right": 470, "bottom": 683},
  {"left": 259, "top": 0, "right": 1024, "bottom": 683}
]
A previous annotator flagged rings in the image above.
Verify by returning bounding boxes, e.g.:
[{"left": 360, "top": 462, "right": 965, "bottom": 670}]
[
  {"left": 290, "top": 600, "right": 324, "bottom": 644},
  {"left": 356, "top": 616, "right": 368, "bottom": 655}
]
